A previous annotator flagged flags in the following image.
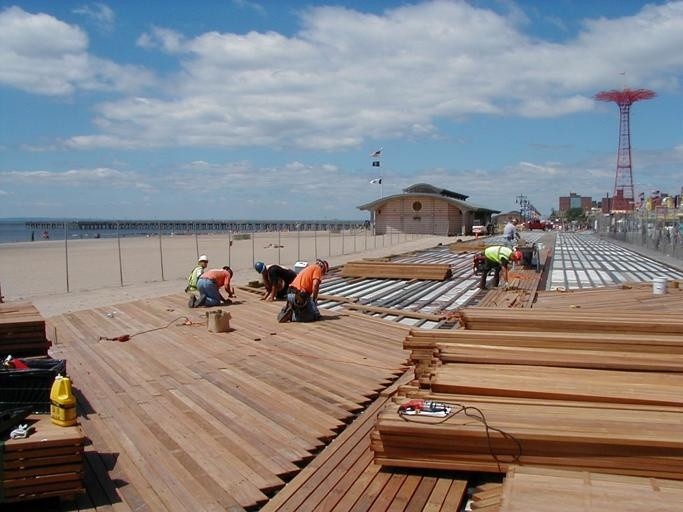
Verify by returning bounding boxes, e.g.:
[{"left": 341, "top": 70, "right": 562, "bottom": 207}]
[
  {"left": 369, "top": 178, "right": 381, "bottom": 185},
  {"left": 367, "top": 150, "right": 384, "bottom": 158},
  {"left": 372, "top": 161, "right": 380, "bottom": 167}
]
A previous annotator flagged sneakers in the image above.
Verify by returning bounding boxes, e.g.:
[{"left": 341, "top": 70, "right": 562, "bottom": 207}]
[
  {"left": 278, "top": 304, "right": 293, "bottom": 322},
  {"left": 481, "top": 287, "right": 488, "bottom": 290},
  {"left": 188, "top": 293, "right": 207, "bottom": 308}
]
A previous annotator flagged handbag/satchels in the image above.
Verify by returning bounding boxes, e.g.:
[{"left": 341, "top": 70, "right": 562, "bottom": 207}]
[{"left": 295, "top": 291, "right": 307, "bottom": 307}]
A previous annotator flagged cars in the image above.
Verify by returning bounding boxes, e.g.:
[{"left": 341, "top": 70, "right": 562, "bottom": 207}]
[{"left": 523, "top": 218, "right": 553, "bottom": 231}]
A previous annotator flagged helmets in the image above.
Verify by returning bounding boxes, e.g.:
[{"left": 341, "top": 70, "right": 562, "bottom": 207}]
[
  {"left": 255, "top": 260, "right": 265, "bottom": 274},
  {"left": 223, "top": 266, "right": 233, "bottom": 279},
  {"left": 512, "top": 219, "right": 518, "bottom": 223},
  {"left": 199, "top": 255, "right": 208, "bottom": 262},
  {"left": 513, "top": 252, "right": 523, "bottom": 264},
  {"left": 316, "top": 259, "right": 328, "bottom": 274}
]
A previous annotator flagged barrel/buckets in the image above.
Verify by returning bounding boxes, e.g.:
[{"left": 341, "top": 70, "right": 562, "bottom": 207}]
[
  {"left": 50, "top": 372, "right": 77, "bottom": 427},
  {"left": 652, "top": 276, "right": 667, "bottom": 294}
]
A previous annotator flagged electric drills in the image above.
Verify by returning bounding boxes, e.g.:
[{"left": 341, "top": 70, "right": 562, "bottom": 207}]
[
  {"left": 400, "top": 399, "right": 446, "bottom": 412},
  {"left": 113, "top": 335, "right": 130, "bottom": 342}
]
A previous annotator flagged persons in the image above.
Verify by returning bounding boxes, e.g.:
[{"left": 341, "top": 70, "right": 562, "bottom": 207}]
[
  {"left": 184, "top": 254, "right": 208, "bottom": 296},
  {"left": 265, "top": 265, "right": 297, "bottom": 302},
  {"left": 254, "top": 262, "right": 288, "bottom": 302},
  {"left": 187, "top": 266, "right": 235, "bottom": 309},
  {"left": 649, "top": 221, "right": 682, "bottom": 257},
  {"left": 276, "top": 259, "right": 329, "bottom": 322},
  {"left": 477, "top": 246, "right": 523, "bottom": 290},
  {"left": 501, "top": 218, "right": 521, "bottom": 246}
]
